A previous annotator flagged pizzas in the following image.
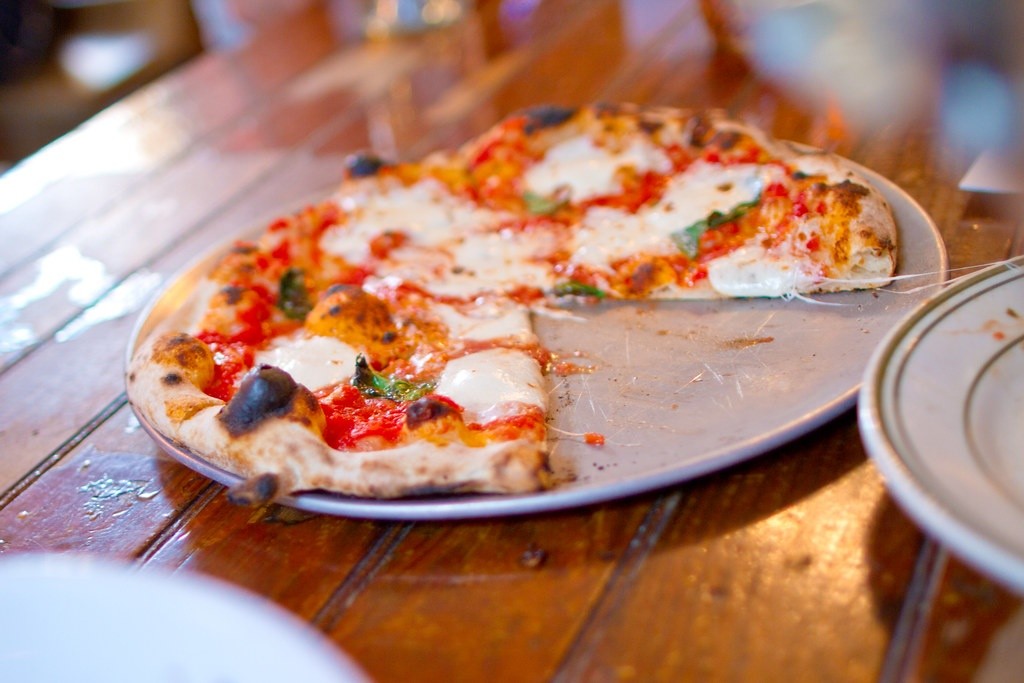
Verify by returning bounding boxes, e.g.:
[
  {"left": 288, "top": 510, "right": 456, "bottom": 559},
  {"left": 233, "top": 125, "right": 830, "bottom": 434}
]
[{"left": 125, "top": 100, "right": 900, "bottom": 503}]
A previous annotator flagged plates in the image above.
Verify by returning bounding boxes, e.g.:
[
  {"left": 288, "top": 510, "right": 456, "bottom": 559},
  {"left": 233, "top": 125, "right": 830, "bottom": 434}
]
[
  {"left": 856, "top": 256, "right": 1024, "bottom": 602},
  {"left": 123, "top": 138, "right": 950, "bottom": 520},
  {"left": 0, "top": 552, "right": 376, "bottom": 683}
]
